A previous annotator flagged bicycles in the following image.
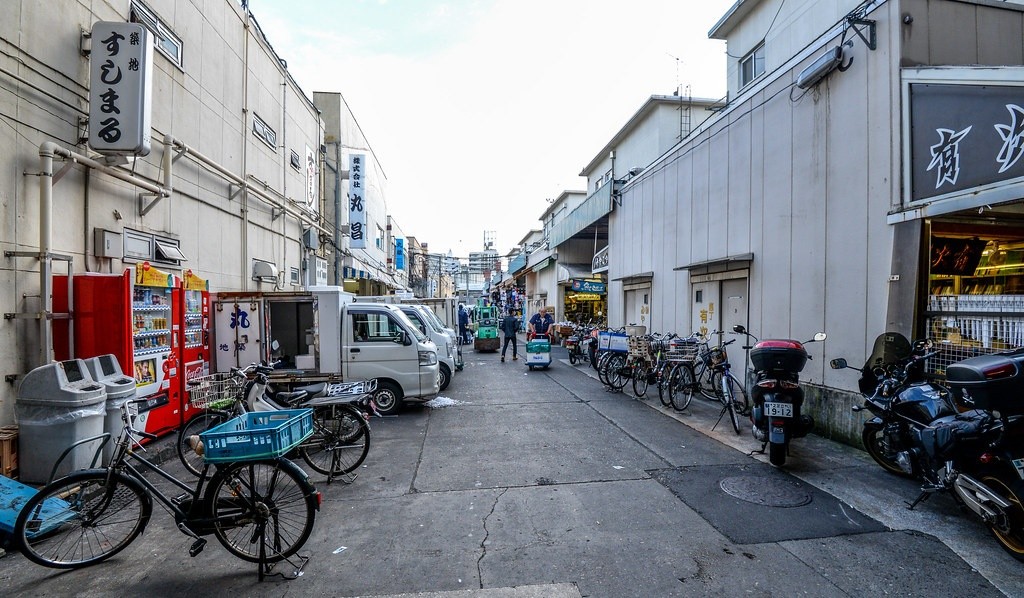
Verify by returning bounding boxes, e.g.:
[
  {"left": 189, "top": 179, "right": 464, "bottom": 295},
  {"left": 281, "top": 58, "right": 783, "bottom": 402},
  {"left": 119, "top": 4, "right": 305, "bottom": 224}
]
[
  {"left": 14, "top": 397, "right": 323, "bottom": 584},
  {"left": 698, "top": 338, "right": 750, "bottom": 435},
  {"left": 174, "top": 339, "right": 384, "bottom": 486},
  {"left": 560, "top": 311, "right": 726, "bottom": 417}
]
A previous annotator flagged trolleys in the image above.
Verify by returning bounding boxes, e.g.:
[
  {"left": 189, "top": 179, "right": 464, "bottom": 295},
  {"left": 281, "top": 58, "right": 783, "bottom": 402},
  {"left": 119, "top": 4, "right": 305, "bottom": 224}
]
[
  {"left": 524, "top": 333, "right": 552, "bottom": 371},
  {"left": 0, "top": 432, "right": 113, "bottom": 542}
]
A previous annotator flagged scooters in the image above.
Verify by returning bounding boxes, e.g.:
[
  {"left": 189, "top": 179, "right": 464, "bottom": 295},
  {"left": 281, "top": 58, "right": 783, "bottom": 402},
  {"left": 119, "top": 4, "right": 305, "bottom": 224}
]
[{"left": 733, "top": 324, "right": 828, "bottom": 468}]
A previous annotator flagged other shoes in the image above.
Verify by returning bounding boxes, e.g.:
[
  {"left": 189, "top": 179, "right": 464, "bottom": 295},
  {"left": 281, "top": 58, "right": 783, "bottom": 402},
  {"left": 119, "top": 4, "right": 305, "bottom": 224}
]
[
  {"left": 513, "top": 356, "right": 518, "bottom": 360},
  {"left": 501, "top": 355, "right": 504, "bottom": 361}
]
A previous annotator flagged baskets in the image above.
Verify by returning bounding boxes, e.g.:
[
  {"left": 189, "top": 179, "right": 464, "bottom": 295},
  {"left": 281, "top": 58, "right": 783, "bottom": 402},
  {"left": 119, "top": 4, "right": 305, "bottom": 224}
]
[
  {"left": 674, "top": 336, "right": 698, "bottom": 356},
  {"left": 650, "top": 339, "right": 671, "bottom": 351},
  {"left": 189, "top": 370, "right": 241, "bottom": 410},
  {"left": 700, "top": 345, "right": 728, "bottom": 366},
  {"left": 663, "top": 345, "right": 698, "bottom": 361}
]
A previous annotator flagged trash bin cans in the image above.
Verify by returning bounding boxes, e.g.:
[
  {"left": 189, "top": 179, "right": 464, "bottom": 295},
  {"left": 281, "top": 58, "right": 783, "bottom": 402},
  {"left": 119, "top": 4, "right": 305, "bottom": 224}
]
[
  {"left": 15, "top": 358, "right": 107, "bottom": 485},
  {"left": 86, "top": 353, "right": 143, "bottom": 463}
]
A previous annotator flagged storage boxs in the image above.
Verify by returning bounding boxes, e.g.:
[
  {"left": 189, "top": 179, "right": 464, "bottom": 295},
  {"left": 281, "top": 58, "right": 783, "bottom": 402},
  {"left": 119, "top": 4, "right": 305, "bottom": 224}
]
[
  {"left": 598, "top": 331, "right": 629, "bottom": 352},
  {"left": 750, "top": 339, "right": 808, "bottom": 372},
  {"left": 945, "top": 347, "right": 1024, "bottom": 410},
  {"left": 526, "top": 339, "right": 552, "bottom": 365},
  {"left": 199, "top": 408, "right": 315, "bottom": 462}
]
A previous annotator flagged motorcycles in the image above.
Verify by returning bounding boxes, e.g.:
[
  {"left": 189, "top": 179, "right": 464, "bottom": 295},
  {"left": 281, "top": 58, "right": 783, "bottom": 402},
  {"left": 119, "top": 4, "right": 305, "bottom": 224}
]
[{"left": 828, "top": 332, "right": 1024, "bottom": 564}]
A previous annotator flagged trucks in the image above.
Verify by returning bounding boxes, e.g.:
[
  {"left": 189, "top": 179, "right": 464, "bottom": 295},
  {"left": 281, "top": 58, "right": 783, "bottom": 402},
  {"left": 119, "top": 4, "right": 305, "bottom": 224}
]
[
  {"left": 308, "top": 285, "right": 501, "bottom": 393},
  {"left": 210, "top": 284, "right": 440, "bottom": 418}
]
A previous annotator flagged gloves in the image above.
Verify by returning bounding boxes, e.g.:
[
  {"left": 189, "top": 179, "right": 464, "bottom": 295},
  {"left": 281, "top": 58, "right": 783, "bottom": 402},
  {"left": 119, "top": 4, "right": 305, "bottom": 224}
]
[
  {"left": 532, "top": 332, "right": 536, "bottom": 338},
  {"left": 545, "top": 332, "right": 549, "bottom": 337}
]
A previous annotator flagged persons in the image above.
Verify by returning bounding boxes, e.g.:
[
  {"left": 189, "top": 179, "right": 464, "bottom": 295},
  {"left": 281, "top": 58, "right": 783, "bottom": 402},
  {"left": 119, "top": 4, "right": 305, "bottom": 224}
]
[
  {"left": 457, "top": 305, "right": 470, "bottom": 345},
  {"left": 468, "top": 307, "right": 477, "bottom": 323},
  {"left": 501, "top": 308, "right": 520, "bottom": 362},
  {"left": 528, "top": 307, "right": 554, "bottom": 340}
]
